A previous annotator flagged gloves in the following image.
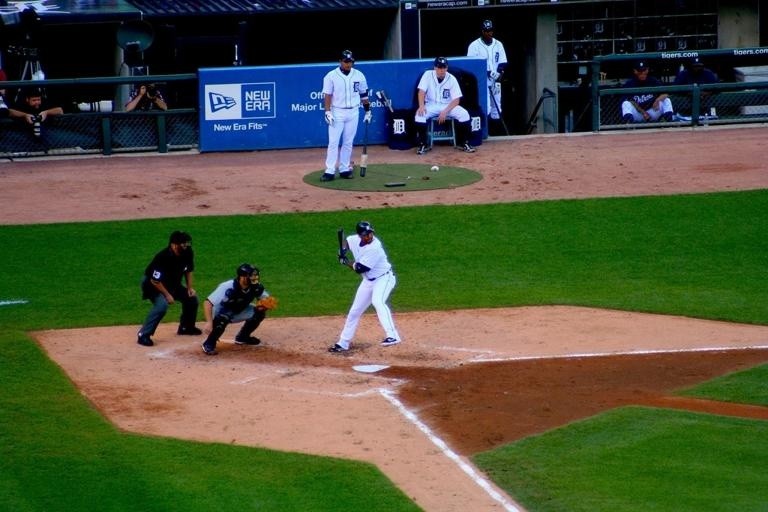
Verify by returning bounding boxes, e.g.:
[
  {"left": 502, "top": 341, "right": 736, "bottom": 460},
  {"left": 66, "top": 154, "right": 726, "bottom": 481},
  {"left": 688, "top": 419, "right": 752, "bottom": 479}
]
[
  {"left": 363, "top": 110, "right": 373, "bottom": 123},
  {"left": 489, "top": 70, "right": 502, "bottom": 81},
  {"left": 324, "top": 112, "right": 335, "bottom": 125},
  {"left": 339, "top": 256, "right": 349, "bottom": 265}
]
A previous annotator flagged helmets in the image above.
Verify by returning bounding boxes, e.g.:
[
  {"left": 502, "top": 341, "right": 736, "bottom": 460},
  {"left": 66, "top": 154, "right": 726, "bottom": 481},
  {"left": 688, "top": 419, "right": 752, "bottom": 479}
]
[
  {"left": 434, "top": 56, "right": 448, "bottom": 68},
  {"left": 692, "top": 57, "right": 704, "bottom": 66},
  {"left": 236, "top": 263, "right": 260, "bottom": 276},
  {"left": 483, "top": 19, "right": 494, "bottom": 32},
  {"left": 340, "top": 50, "right": 355, "bottom": 63},
  {"left": 633, "top": 59, "right": 650, "bottom": 70},
  {"left": 356, "top": 222, "right": 374, "bottom": 237}
]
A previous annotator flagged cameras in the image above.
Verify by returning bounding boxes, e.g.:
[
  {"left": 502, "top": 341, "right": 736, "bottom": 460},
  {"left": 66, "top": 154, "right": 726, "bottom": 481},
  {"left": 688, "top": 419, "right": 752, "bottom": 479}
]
[
  {"left": 145, "top": 86, "right": 157, "bottom": 97},
  {"left": 32, "top": 115, "right": 42, "bottom": 142}
]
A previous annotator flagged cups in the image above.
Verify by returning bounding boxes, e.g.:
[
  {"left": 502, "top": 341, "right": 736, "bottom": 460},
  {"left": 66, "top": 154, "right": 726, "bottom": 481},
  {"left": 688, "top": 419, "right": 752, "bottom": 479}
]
[{"left": 576, "top": 78, "right": 582, "bottom": 85}]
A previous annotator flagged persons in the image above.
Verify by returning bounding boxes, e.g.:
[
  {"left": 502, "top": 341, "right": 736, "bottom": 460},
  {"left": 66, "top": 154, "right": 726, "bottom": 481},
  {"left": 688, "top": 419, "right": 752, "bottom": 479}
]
[
  {"left": 675, "top": 56, "right": 720, "bottom": 120},
  {"left": 319, "top": 50, "right": 373, "bottom": 182},
  {"left": 619, "top": 60, "right": 678, "bottom": 128},
  {"left": 327, "top": 219, "right": 401, "bottom": 353},
  {"left": 201, "top": 264, "right": 279, "bottom": 356},
  {"left": 466, "top": 18, "right": 509, "bottom": 136},
  {"left": 137, "top": 231, "right": 202, "bottom": 346},
  {"left": 414, "top": 57, "right": 476, "bottom": 155},
  {"left": 0, "top": 65, "right": 168, "bottom": 153}
]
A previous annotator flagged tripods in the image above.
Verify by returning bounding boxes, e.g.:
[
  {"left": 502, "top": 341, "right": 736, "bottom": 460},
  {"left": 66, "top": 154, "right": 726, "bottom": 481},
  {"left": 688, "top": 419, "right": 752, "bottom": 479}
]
[{"left": 12, "top": 59, "right": 48, "bottom": 104}]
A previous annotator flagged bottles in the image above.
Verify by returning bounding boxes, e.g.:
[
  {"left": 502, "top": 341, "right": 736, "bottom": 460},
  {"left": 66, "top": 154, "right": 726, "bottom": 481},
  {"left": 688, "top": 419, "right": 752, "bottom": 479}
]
[
  {"left": 703, "top": 112, "right": 709, "bottom": 128},
  {"left": 680, "top": 64, "right": 684, "bottom": 73}
]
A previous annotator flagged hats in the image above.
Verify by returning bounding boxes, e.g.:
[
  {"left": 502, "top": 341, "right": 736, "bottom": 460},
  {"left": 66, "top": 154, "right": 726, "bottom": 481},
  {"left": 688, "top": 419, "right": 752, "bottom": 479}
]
[{"left": 169, "top": 231, "right": 192, "bottom": 244}]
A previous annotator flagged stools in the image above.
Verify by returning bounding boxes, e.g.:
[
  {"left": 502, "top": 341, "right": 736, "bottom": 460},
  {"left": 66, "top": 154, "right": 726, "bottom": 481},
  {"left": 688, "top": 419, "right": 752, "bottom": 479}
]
[{"left": 426, "top": 116, "right": 457, "bottom": 150}]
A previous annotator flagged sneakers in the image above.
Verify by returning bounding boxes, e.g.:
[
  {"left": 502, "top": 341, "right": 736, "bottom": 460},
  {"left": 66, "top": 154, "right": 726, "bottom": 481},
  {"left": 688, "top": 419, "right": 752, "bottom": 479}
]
[
  {"left": 137, "top": 334, "right": 153, "bottom": 346},
  {"left": 416, "top": 144, "right": 427, "bottom": 154},
  {"left": 235, "top": 335, "right": 260, "bottom": 345},
  {"left": 329, "top": 344, "right": 344, "bottom": 352},
  {"left": 320, "top": 172, "right": 335, "bottom": 181},
  {"left": 463, "top": 141, "right": 477, "bottom": 152},
  {"left": 202, "top": 339, "right": 216, "bottom": 354},
  {"left": 339, "top": 171, "right": 353, "bottom": 179},
  {"left": 381, "top": 337, "right": 401, "bottom": 347},
  {"left": 178, "top": 326, "right": 202, "bottom": 335}
]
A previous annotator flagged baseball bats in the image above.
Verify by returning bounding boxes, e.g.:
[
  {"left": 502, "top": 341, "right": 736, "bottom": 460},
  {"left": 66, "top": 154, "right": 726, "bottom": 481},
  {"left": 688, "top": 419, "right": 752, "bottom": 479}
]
[
  {"left": 360, "top": 121, "right": 368, "bottom": 177},
  {"left": 376, "top": 91, "right": 393, "bottom": 113},
  {"left": 338, "top": 228, "right": 344, "bottom": 258},
  {"left": 489, "top": 86, "right": 509, "bottom": 135}
]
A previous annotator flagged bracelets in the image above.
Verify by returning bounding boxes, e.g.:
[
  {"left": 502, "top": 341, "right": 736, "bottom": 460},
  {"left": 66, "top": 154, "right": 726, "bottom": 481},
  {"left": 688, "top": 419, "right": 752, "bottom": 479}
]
[{"left": 363, "top": 103, "right": 369, "bottom": 111}]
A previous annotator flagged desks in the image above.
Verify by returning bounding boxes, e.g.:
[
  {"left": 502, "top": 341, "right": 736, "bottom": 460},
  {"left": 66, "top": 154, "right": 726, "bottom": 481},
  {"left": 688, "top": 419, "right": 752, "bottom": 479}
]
[{"left": 557, "top": 74, "right": 675, "bottom": 131}]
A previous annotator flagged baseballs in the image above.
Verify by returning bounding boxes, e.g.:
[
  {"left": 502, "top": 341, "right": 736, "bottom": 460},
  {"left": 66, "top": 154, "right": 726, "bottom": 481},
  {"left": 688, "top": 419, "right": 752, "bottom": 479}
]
[{"left": 431, "top": 166, "right": 439, "bottom": 170}]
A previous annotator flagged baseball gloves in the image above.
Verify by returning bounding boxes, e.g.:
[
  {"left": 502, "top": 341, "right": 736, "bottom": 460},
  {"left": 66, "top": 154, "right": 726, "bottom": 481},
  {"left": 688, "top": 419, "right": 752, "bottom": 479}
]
[{"left": 256, "top": 297, "right": 276, "bottom": 312}]
